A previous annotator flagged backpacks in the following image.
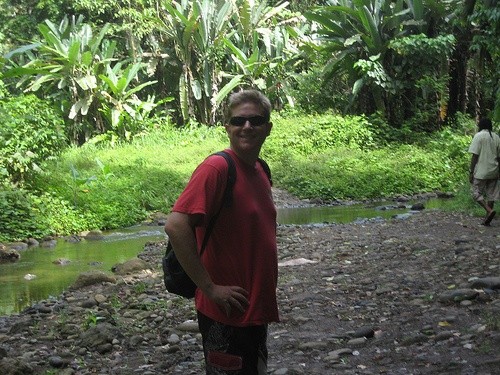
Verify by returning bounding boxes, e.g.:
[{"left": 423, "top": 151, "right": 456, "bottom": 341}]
[{"left": 162, "top": 150, "right": 274, "bottom": 300}]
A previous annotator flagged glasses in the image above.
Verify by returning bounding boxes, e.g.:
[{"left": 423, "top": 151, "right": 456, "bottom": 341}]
[{"left": 228, "top": 116, "right": 267, "bottom": 126}]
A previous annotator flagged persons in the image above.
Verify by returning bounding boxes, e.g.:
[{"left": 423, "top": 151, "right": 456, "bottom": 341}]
[
  {"left": 468, "top": 117, "right": 500, "bottom": 226},
  {"left": 163, "top": 88, "right": 280, "bottom": 375}
]
